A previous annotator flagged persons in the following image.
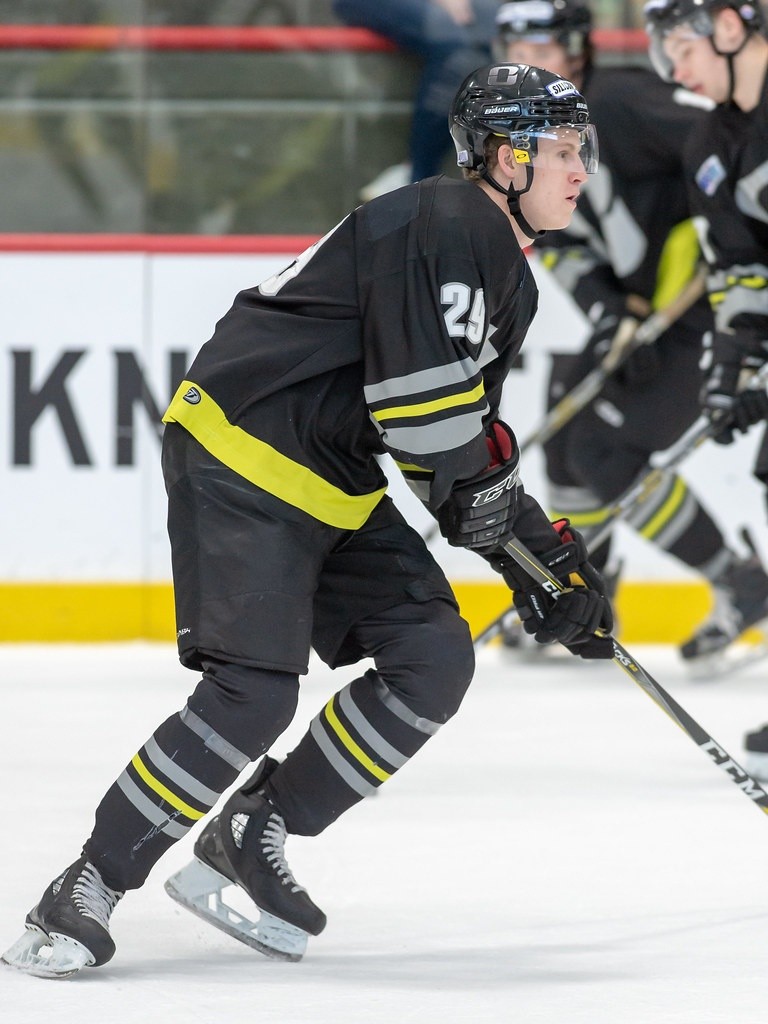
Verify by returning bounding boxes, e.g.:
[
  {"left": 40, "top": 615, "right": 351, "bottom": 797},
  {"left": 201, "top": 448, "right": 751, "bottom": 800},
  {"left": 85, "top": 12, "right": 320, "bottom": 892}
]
[
  {"left": 497, "top": 0, "right": 768, "bottom": 688},
  {"left": 0, "top": 64, "right": 615, "bottom": 979}
]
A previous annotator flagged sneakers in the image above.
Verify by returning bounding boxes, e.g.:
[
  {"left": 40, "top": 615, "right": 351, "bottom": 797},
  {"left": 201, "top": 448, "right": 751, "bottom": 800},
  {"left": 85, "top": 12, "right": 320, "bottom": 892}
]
[
  {"left": 166, "top": 756, "right": 327, "bottom": 960},
  {"left": 676, "top": 529, "right": 768, "bottom": 687},
  {"left": 0, "top": 854, "right": 125, "bottom": 979}
]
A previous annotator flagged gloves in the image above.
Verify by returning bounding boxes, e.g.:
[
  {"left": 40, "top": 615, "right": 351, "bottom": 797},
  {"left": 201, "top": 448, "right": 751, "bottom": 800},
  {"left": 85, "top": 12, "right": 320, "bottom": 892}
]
[
  {"left": 696, "top": 330, "right": 768, "bottom": 444},
  {"left": 503, "top": 519, "right": 616, "bottom": 660},
  {"left": 426, "top": 415, "right": 521, "bottom": 551}
]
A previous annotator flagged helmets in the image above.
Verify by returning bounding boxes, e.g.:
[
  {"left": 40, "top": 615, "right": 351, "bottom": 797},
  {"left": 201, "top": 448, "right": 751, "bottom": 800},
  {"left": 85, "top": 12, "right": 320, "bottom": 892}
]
[
  {"left": 490, "top": 0, "right": 593, "bottom": 99},
  {"left": 447, "top": 63, "right": 600, "bottom": 177},
  {"left": 642, "top": 0, "right": 762, "bottom": 84}
]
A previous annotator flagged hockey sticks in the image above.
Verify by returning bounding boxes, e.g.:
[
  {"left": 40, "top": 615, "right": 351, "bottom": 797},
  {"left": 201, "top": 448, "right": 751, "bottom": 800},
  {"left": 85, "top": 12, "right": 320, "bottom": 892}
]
[
  {"left": 472, "top": 360, "right": 768, "bottom": 643},
  {"left": 501, "top": 533, "right": 768, "bottom": 812},
  {"left": 414, "top": 260, "right": 712, "bottom": 543}
]
